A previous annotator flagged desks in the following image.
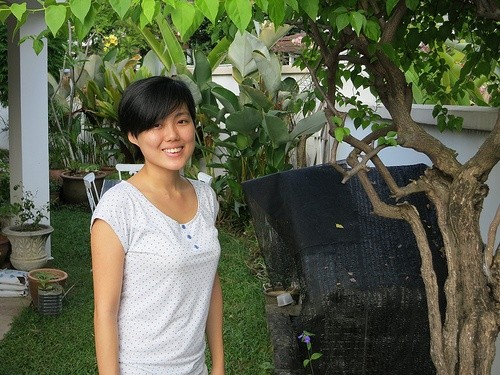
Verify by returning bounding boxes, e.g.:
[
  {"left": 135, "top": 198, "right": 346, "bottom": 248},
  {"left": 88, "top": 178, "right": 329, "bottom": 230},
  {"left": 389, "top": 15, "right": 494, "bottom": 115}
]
[{"left": 100, "top": 179, "right": 122, "bottom": 199}]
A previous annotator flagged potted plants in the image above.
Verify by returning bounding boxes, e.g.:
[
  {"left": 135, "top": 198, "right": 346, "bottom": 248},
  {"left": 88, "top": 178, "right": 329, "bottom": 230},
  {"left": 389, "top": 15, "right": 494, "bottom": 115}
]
[
  {"left": 37, "top": 273, "right": 63, "bottom": 316},
  {"left": 48, "top": 98, "right": 74, "bottom": 185},
  {"left": 28, "top": 268, "right": 68, "bottom": 307},
  {"left": 60, "top": 159, "right": 107, "bottom": 204},
  {"left": 0, "top": 183, "right": 60, "bottom": 272}
]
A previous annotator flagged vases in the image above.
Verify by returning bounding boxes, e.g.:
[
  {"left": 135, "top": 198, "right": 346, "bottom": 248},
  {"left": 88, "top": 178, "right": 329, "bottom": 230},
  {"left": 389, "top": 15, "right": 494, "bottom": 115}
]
[{"left": 0, "top": 234, "right": 9, "bottom": 267}]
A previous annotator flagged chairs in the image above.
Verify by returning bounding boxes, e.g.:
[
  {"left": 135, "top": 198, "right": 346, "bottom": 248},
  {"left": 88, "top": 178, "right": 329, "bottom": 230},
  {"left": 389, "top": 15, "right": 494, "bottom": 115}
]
[
  {"left": 83, "top": 172, "right": 100, "bottom": 214},
  {"left": 115, "top": 163, "right": 145, "bottom": 180},
  {"left": 197, "top": 171, "right": 213, "bottom": 187}
]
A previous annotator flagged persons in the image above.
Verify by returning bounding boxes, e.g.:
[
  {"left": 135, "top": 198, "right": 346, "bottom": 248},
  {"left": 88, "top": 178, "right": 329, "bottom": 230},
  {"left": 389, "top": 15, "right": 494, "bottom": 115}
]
[{"left": 90, "top": 76, "right": 224, "bottom": 375}]
